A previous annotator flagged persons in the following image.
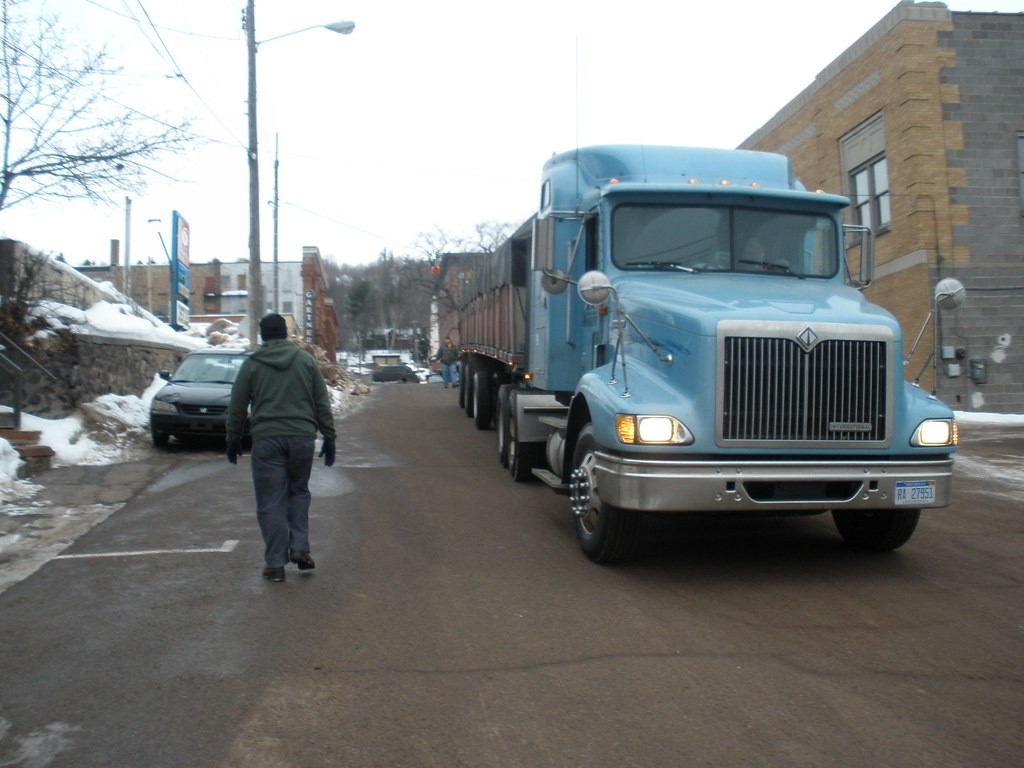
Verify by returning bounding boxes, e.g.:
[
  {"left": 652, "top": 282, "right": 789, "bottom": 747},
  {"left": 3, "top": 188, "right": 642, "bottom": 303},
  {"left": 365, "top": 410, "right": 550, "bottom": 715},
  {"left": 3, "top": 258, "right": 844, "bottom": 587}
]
[
  {"left": 743, "top": 237, "right": 791, "bottom": 271},
  {"left": 435, "top": 336, "right": 460, "bottom": 389},
  {"left": 224, "top": 312, "right": 338, "bottom": 585}
]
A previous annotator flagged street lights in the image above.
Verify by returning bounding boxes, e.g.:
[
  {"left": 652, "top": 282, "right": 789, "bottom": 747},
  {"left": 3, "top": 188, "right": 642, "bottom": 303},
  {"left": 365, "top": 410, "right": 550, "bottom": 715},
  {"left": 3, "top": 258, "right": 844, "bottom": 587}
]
[{"left": 246, "top": 19, "right": 356, "bottom": 340}]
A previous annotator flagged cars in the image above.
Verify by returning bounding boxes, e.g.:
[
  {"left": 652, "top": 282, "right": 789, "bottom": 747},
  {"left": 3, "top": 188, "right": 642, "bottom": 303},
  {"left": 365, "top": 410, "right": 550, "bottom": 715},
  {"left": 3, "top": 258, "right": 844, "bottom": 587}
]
[{"left": 149, "top": 346, "right": 257, "bottom": 450}]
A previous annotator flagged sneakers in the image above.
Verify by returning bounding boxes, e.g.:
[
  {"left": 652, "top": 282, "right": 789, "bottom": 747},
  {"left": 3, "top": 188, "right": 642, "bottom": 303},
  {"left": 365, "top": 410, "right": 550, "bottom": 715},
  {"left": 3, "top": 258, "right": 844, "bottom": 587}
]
[
  {"left": 262, "top": 565, "right": 287, "bottom": 582},
  {"left": 290, "top": 547, "right": 315, "bottom": 569}
]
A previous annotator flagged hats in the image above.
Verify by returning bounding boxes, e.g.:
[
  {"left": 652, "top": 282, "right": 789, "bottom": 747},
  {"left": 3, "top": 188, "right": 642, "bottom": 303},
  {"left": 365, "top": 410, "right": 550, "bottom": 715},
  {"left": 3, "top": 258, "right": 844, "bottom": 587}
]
[{"left": 258, "top": 314, "right": 287, "bottom": 341}]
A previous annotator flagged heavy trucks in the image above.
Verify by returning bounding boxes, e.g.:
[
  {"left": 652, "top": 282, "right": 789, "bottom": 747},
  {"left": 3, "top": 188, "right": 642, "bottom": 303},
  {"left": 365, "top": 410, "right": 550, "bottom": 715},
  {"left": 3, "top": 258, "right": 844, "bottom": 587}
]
[{"left": 455, "top": 143, "right": 970, "bottom": 566}]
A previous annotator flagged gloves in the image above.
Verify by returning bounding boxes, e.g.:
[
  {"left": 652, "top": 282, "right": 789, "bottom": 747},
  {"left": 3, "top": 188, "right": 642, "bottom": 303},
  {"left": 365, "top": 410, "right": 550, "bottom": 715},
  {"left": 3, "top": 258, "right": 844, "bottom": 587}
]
[
  {"left": 318, "top": 437, "right": 337, "bottom": 467},
  {"left": 226, "top": 438, "right": 244, "bottom": 465}
]
